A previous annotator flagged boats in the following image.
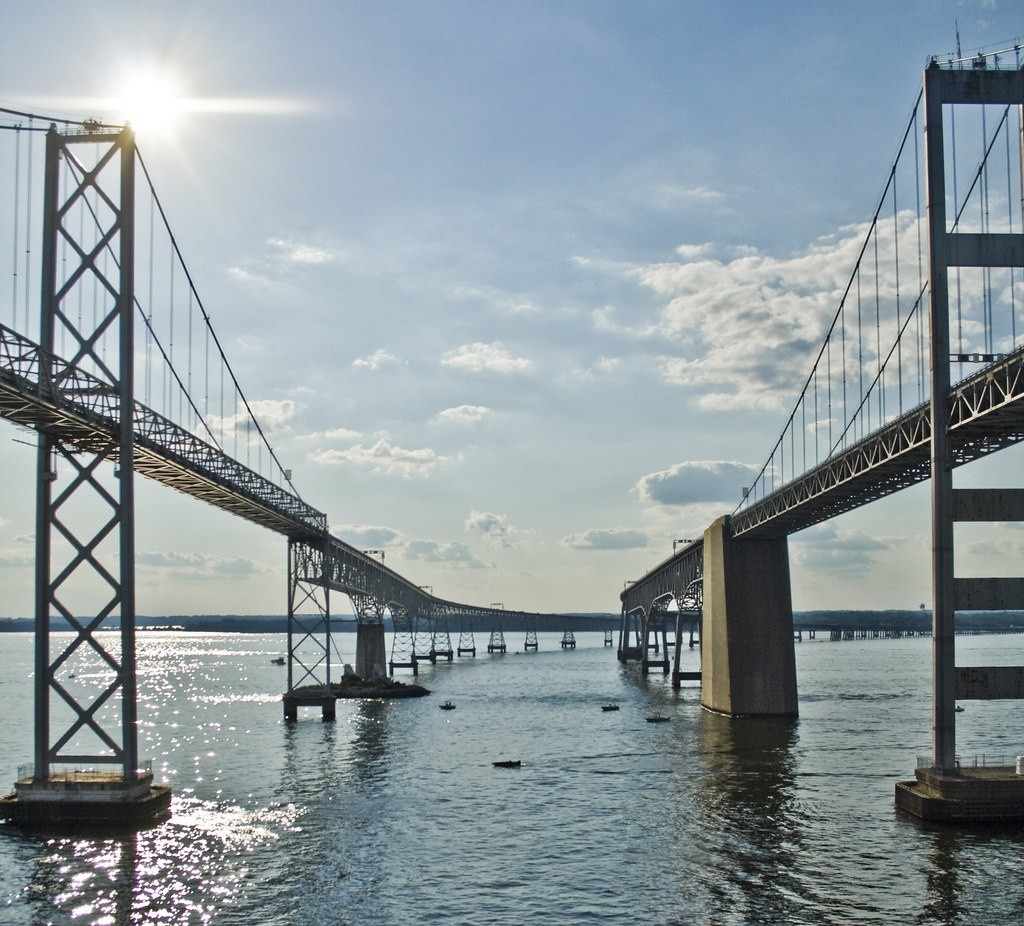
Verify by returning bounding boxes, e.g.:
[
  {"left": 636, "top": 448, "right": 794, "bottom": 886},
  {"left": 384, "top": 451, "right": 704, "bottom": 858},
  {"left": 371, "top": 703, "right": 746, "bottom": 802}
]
[
  {"left": 600, "top": 704, "right": 620, "bottom": 711},
  {"left": 645, "top": 712, "right": 670, "bottom": 723},
  {"left": 492, "top": 760, "right": 521, "bottom": 767},
  {"left": 271, "top": 657, "right": 286, "bottom": 666},
  {"left": 439, "top": 701, "right": 455, "bottom": 710}
]
